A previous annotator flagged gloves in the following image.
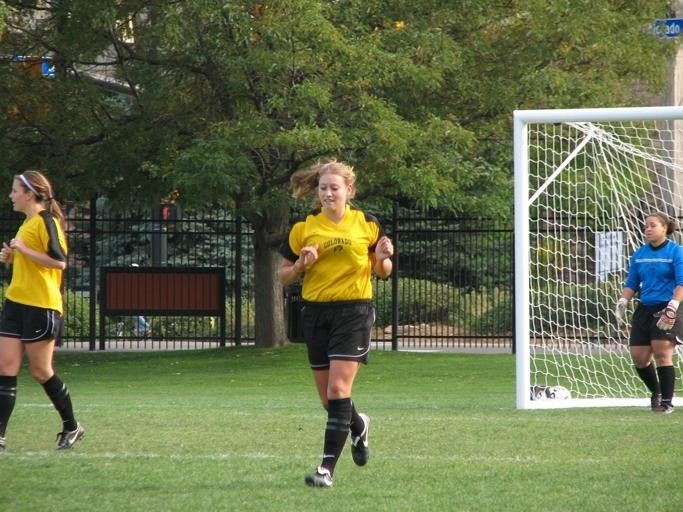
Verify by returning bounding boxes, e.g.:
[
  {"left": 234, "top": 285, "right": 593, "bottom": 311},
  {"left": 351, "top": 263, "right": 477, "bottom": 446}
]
[
  {"left": 615, "top": 298, "right": 628, "bottom": 319},
  {"left": 653, "top": 309, "right": 676, "bottom": 331}
]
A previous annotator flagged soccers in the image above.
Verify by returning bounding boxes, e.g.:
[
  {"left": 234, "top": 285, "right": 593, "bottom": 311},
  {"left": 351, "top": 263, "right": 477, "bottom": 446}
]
[{"left": 548, "top": 384, "right": 571, "bottom": 400}]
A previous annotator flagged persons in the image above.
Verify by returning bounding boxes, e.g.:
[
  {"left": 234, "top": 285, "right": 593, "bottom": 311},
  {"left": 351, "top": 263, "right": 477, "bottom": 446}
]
[
  {"left": 116, "top": 317, "right": 124, "bottom": 336},
  {"left": 0, "top": 170, "right": 84, "bottom": 450},
  {"left": 613, "top": 212, "right": 683, "bottom": 413},
  {"left": 278, "top": 161, "right": 394, "bottom": 488},
  {"left": 145, "top": 319, "right": 151, "bottom": 337},
  {"left": 134, "top": 328, "right": 144, "bottom": 336}
]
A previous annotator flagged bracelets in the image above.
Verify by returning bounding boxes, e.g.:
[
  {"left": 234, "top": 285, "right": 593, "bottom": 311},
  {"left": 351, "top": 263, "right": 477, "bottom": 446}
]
[
  {"left": 617, "top": 298, "right": 628, "bottom": 306},
  {"left": 667, "top": 299, "right": 679, "bottom": 312}
]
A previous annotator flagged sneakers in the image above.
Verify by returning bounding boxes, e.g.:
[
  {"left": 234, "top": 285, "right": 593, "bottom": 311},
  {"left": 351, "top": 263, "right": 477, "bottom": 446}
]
[
  {"left": 55, "top": 422, "right": 85, "bottom": 450},
  {"left": 350, "top": 413, "right": 370, "bottom": 466},
  {"left": 654, "top": 401, "right": 673, "bottom": 414},
  {"left": 0, "top": 436, "right": 6, "bottom": 447},
  {"left": 651, "top": 394, "right": 663, "bottom": 409},
  {"left": 305, "top": 466, "right": 333, "bottom": 488}
]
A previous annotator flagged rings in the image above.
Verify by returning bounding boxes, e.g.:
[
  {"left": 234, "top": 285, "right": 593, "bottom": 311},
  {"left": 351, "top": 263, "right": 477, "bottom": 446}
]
[{"left": 11, "top": 238, "right": 14, "bottom": 241}]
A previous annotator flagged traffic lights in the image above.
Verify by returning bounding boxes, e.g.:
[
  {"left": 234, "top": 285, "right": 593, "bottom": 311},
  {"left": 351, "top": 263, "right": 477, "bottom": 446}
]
[{"left": 160, "top": 203, "right": 181, "bottom": 222}]
